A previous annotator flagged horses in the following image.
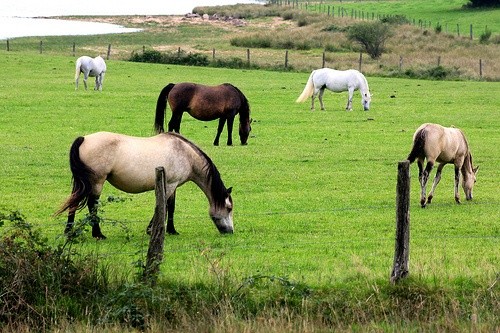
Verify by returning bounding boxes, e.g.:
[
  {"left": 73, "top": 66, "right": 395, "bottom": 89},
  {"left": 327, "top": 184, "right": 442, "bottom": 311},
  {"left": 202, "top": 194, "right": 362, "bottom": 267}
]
[
  {"left": 296, "top": 67, "right": 373, "bottom": 111},
  {"left": 75, "top": 56, "right": 106, "bottom": 91},
  {"left": 407, "top": 123, "right": 479, "bottom": 208},
  {"left": 49, "top": 131, "right": 234, "bottom": 240},
  {"left": 153, "top": 82, "right": 253, "bottom": 146}
]
